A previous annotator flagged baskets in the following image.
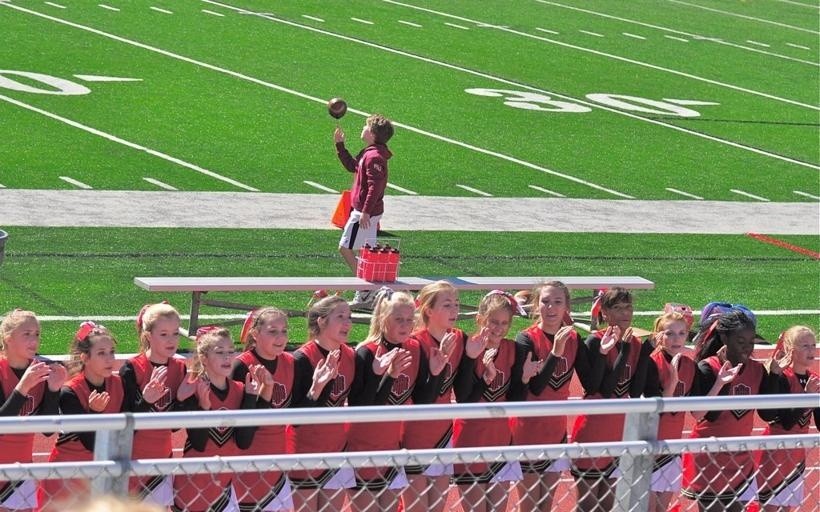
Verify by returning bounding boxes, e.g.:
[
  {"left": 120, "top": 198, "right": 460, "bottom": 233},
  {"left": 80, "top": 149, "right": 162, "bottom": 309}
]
[{"left": 355, "top": 238, "right": 402, "bottom": 284}]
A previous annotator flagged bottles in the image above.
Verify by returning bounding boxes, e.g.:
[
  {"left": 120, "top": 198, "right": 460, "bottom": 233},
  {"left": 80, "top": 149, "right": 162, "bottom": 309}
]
[{"left": 357, "top": 244, "right": 399, "bottom": 283}]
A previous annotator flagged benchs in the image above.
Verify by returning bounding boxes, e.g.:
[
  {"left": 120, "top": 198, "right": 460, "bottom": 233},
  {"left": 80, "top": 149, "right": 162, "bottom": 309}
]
[{"left": 132, "top": 274, "right": 655, "bottom": 343}]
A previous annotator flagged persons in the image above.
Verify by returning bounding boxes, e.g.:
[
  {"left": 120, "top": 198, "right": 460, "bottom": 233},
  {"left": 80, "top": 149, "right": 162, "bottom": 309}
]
[
  {"left": 334, "top": 114, "right": 394, "bottom": 310},
  {"left": 1, "top": 283, "right": 820, "bottom": 512}
]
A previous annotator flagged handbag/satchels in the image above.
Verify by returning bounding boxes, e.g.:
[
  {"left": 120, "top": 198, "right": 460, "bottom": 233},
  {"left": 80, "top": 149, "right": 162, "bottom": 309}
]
[
  {"left": 700, "top": 302, "right": 756, "bottom": 337},
  {"left": 663, "top": 302, "right": 694, "bottom": 331}
]
